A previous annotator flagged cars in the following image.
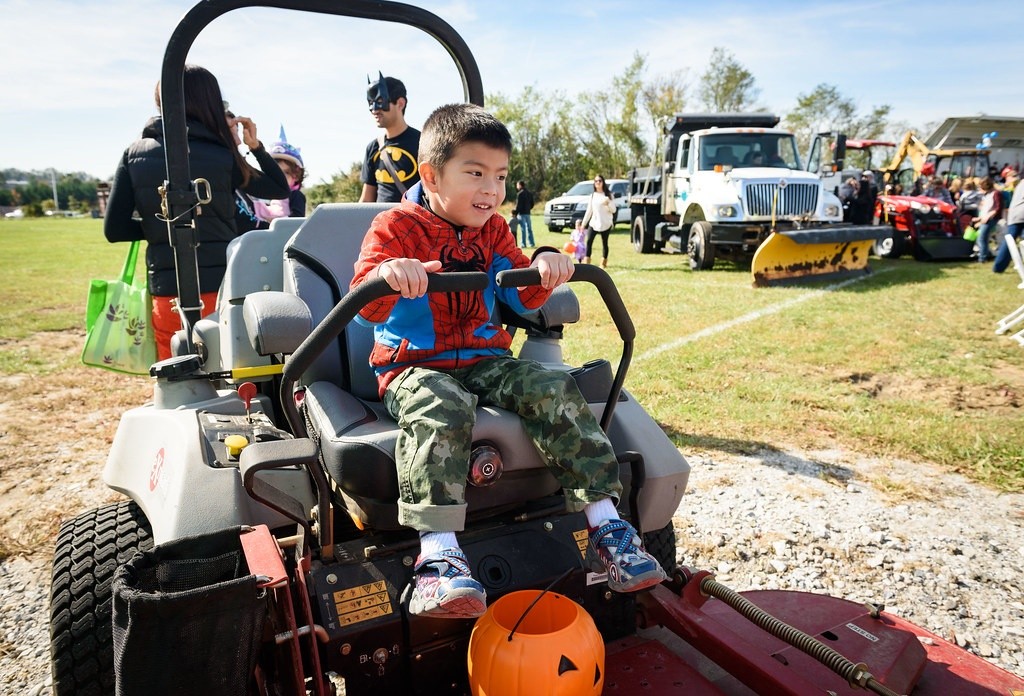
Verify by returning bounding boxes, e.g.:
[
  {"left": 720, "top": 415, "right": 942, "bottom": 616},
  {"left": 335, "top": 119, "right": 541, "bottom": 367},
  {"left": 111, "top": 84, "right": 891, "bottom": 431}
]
[{"left": 4, "top": 208, "right": 25, "bottom": 218}]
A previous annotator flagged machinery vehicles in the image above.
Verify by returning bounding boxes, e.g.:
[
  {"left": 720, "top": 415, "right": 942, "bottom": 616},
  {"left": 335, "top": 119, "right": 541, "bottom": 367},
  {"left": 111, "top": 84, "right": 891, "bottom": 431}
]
[
  {"left": 807, "top": 132, "right": 976, "bottom": 260},
  {"left": 623, "top": 112, "right": 896, "bottom": 287},
  {"left": 880, "top": 129, "right": 1002, "bottom": 204}
]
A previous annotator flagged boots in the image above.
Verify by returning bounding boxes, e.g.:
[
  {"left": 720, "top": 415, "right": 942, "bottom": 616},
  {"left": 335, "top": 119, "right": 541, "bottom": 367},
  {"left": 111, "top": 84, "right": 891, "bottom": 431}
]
[
  {"left": 584, "top": 257, "right": 591, "bottom": 264},
  {"left": 600, "top": 258, "right": 608, "bottom": 269}
]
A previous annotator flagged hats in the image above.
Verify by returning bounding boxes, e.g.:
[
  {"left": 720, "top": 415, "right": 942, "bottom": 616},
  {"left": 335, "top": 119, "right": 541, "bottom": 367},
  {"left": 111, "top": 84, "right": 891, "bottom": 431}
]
[{"left": 267, "top": 123, "right": 305, "bottom": 169}]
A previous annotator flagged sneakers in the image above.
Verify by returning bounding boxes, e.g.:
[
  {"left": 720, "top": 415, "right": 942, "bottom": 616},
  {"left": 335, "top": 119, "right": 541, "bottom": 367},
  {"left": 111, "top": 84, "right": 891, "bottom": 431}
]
[
  {"left": 404, "top": 546, "right": 488, "bottom": 619},
  {"left": 585, "top": 518, "right": 667, "bottom": 592}
]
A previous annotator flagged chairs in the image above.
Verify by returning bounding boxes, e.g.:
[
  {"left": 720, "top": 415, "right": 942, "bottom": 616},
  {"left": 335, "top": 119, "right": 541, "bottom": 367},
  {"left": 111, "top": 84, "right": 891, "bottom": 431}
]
[{"left": 242, "top": 201, "right": 580, "bottom": 530}]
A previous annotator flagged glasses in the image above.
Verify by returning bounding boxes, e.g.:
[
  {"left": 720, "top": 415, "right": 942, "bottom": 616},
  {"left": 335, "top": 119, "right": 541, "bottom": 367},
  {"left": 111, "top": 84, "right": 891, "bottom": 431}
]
[
  {"left": 595, "top": 179, "right": 602, "bottom": 183},
  {"left": 861, "top": 175, "right": 868, "bottom": 178}
]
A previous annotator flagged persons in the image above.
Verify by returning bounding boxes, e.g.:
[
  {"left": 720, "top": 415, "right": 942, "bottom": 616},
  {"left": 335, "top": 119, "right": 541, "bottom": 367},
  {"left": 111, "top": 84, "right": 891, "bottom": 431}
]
[
  {"left": 348, "top": 103, "right": 668, "bottom": 618},
  {"left": 752, "top": 152, "right": 763, "bottom": 165},
  {"left": 569, "top": 219, "right": 586, "bottom": 263},
  {"left": 515, "top": 180, "right": 535, "bottom": 247},
  {"left": 888, "top": 161, "right": 1024, "bottom": 273},
  {"left": 837, "top": 169, "right": 878, "bottom": 225},
  {"left": 580, "top": 174, "right": 616, "bottom": 268},
  {"left": 508, "top": 210, "right": 521, "bottom": 247},
  {"left": 357, "top": 71, "right": 423, "bottom": 202},
  {"left": 103, "top": 64, "right": 306, "bottom": 362}
]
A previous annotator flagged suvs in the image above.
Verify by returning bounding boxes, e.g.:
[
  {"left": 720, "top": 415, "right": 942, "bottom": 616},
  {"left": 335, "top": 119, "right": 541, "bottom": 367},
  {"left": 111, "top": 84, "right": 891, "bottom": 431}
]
[{"left": 544, "top": 177, "right": 631, "bottom": 234}]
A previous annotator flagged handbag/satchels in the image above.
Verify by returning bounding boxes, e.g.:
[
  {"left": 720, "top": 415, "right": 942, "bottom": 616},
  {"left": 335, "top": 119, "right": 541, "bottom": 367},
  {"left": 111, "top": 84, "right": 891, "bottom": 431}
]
[{"left": 81, "top": 239, "right": 156, "bottom": 377}]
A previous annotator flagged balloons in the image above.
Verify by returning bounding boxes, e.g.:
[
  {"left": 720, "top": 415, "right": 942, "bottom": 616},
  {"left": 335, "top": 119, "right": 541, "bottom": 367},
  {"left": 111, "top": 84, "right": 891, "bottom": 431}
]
[
  {"left": 975, "top": 131, "right": 999, "bottom": 149},
  {"left": 563, "top": 242, "right": 578, "bottom": 253}
]
